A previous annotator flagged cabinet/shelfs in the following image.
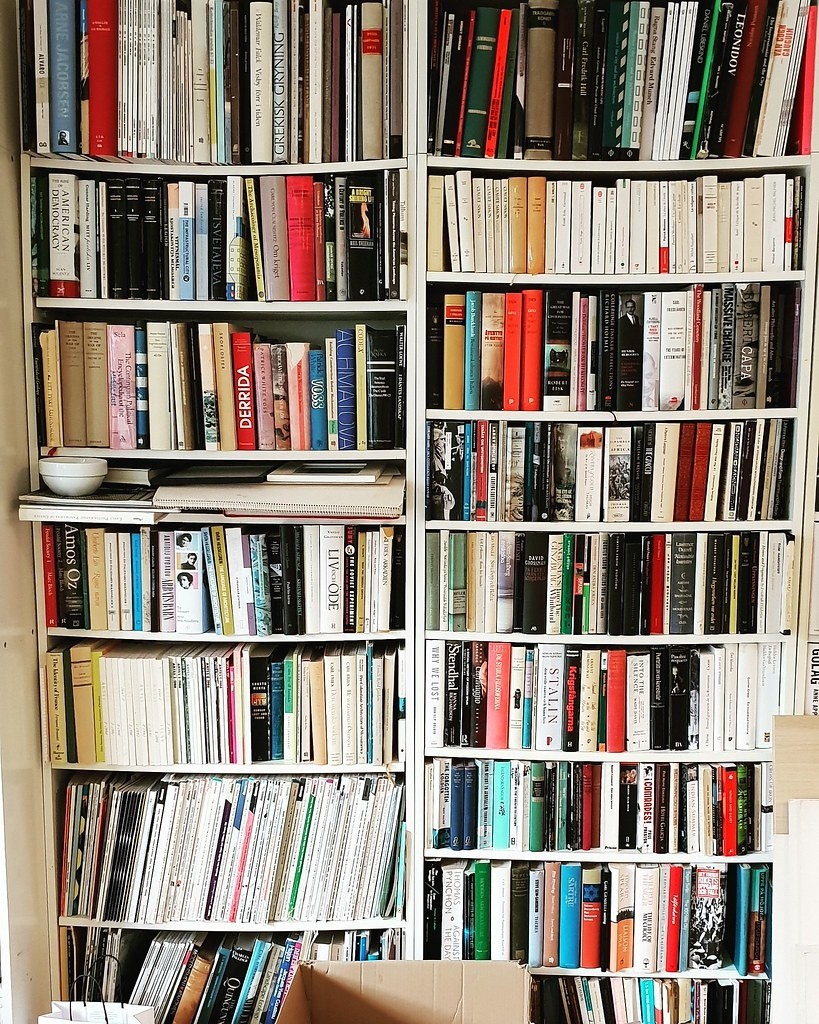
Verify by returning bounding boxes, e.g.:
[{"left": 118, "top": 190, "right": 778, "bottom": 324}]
[
  {"left": 415, "top": 1, "right": 819, "bottom": 1024},
  {"left": 11, "top": 3, "right": 413, "bottom": 1023}
]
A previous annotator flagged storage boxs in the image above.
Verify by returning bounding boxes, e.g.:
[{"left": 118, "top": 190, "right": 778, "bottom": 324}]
[
  {"left": 771, "top": 713, "right": 819, "bottom": 1024},
  {"left": 275, "top": 960, "right": 536, "bottom": 1024}
]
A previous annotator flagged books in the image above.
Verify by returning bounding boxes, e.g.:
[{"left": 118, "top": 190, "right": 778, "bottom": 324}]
[
  {"left": 54, "top": 760, "right": 708, "bottom": 1024},
  {"left": 43, "top": 642, "right": 810, "bottom": 768},
  {"left": 17, "top": 524, "right": 798, "bottom": 638},
  {"left": 15, "top": 0, "right": 817, "bottom": 163},
  {"left": 8, "top": 282, "right": 799, "bottom": 526},
  {"left": 26, "top": 167, "right": 812, "bottom": 305}
]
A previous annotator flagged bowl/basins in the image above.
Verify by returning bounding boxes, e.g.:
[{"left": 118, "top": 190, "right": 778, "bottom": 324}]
[{"left": 38, "top": 457, "right": 108, "bottom": 496}]
